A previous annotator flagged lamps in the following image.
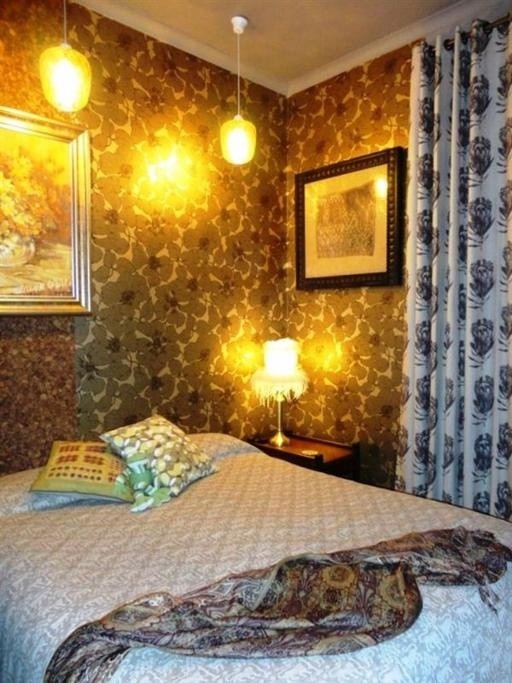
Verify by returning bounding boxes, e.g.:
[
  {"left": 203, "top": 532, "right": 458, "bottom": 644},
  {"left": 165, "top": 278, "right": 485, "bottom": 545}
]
[
  {"left": 39, "top": 1, "right": 92, "bottom": 112},
  {"left": 220, "top": 16, "right": 257, "bottom": 164},
  {"left": 250, "top": 338, "right": 308, "bottom": 447}
]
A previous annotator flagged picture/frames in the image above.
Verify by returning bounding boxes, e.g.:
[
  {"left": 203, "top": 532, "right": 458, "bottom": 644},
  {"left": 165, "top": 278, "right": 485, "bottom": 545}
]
[
  {"left": 294, "top": 146, "right": 405, "bottom": 291},
  {"left": 0, "top": 105, "right": 93, "bottom": 315}
]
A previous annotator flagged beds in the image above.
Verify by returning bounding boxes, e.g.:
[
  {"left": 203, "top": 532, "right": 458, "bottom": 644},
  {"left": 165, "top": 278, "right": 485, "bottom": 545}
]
[{"left": 0, "top": 452, "right": 512, "bottom": 683}]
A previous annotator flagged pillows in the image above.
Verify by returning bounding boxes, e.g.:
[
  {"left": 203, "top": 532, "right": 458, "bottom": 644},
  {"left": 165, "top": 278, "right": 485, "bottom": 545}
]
[
  {"left": 30, "top": 441, "right": 135, "bottom": 504},
  {"left": 186, "top": 432, "right": 265, "bottom": 465},
  {"left": 98, "top": 414, "right": 220, "bottom": 498}
]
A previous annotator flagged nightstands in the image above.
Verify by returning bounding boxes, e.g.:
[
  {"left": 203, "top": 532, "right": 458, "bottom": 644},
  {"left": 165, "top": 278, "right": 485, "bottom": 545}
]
[{"left": 249, "top": 434, "right": 362, "bottom": 482}]
